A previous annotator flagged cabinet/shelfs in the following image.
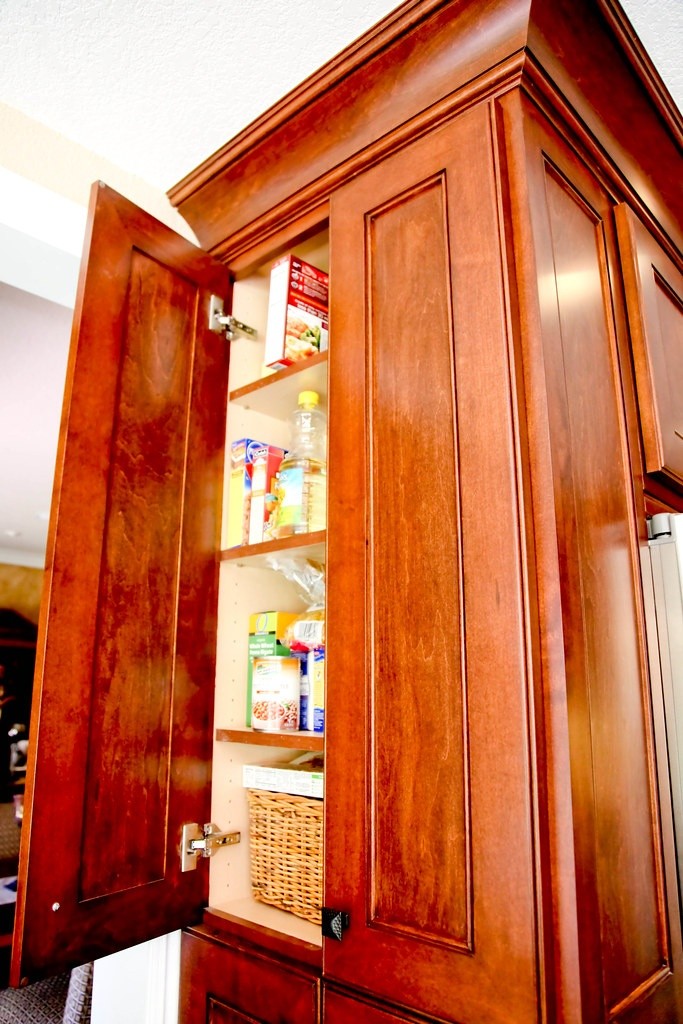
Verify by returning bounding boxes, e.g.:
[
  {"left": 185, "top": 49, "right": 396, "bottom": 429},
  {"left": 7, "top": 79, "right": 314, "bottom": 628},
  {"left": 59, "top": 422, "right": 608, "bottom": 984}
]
[{"left": 6, "top": 1, "right": 683, "bottom": 1023}]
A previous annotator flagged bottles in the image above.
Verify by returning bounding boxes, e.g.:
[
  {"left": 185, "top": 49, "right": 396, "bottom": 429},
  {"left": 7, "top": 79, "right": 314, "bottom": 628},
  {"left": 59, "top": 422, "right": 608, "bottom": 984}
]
[
  {"left": 274, "top": 458, "right": 327, "bottom": 537},
  {"left": 272, "top": 390, "right": 330, "bottom": 464}
]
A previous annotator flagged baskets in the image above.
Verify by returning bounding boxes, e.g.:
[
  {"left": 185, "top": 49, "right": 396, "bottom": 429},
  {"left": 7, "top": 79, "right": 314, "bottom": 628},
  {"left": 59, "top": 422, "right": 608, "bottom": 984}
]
[{"left": 244, "top": 760, "right": 325, "bottom": 926}]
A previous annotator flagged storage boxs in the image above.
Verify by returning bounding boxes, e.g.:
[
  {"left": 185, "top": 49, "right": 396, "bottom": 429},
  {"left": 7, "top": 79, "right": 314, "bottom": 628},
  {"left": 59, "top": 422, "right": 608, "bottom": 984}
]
[
  {"left": 243, "top": 759, "right": 323, "bottom": 799},
  {"left": 245, "top": 610, "right": 309, "bottom": 733}
]
